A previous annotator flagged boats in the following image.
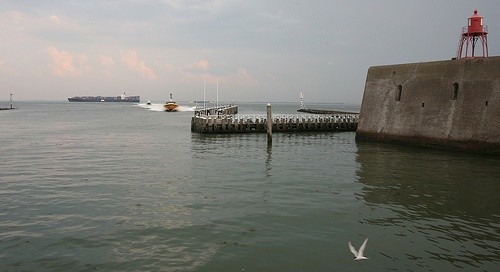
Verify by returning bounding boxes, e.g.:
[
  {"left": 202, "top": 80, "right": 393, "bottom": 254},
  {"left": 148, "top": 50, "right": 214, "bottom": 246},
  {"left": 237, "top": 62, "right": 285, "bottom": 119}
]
[
  {"left": 164, "top": 93, "right": 177, "bottom": 112},
  {"left": 146, "top": 100, "right": 151, "bottom": 105},
  {"left": 192, "top": 100, "right": 211, "bottom": 103}
]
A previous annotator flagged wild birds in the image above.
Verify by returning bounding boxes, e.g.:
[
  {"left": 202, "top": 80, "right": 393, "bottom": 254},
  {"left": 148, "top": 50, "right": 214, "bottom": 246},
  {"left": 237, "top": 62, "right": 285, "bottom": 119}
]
[{"left": 347, "top": 238, "right": 371, "bottom": 261}]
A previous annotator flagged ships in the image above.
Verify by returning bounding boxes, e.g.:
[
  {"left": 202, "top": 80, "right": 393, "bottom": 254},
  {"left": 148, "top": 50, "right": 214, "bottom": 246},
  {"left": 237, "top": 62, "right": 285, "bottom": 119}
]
[{"left": 68, "top": 96, "right": 140, "bottom": 103}]
